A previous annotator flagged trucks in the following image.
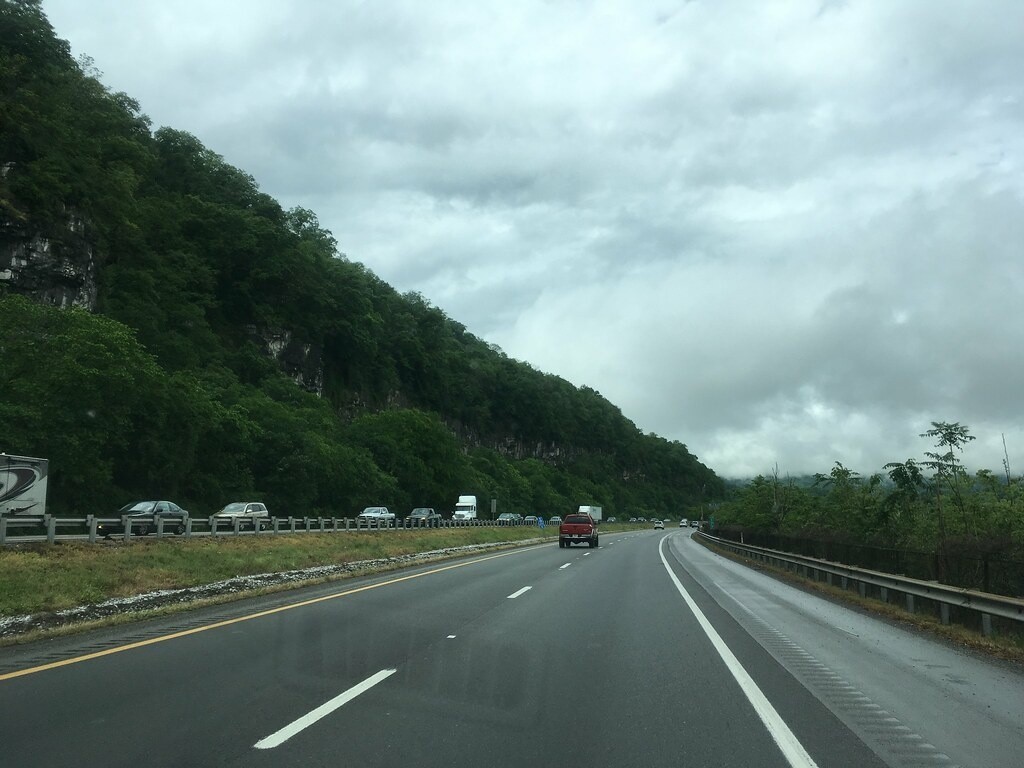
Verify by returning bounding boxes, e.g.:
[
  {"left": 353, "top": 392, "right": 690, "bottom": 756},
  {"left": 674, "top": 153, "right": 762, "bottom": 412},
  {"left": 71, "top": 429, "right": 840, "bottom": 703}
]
[
  {"left": 576, "top": 505, "right": 603, "bottom": 524},
  {"left": 0, "top": 452, "right": 49, "bottom": 516}
]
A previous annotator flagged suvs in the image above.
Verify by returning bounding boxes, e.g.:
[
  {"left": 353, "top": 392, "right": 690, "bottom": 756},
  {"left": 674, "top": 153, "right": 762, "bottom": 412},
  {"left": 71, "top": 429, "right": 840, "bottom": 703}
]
[
  {"left": 654, "top": 521, "right": 664, "bottom": 530},
  {"left": 679, "top": 519, "right": 699, "bottom": 528},
  {"left": 209, "top": 502, "right": 268, "bottom": 531}
]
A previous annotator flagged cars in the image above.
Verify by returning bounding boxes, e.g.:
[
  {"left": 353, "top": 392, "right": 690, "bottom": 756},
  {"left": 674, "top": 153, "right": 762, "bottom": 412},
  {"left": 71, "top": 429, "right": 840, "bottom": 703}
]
[
  {"left": 606, "top": 517, "right": 616, "bottom": 523},
  {"left": 94, "top": 500, "right": 189, "bottom": 536},
  {"left": 629, "top": 517, "right": 671, "bottom": 522},
  {"left": 549, "top": 517, "right": 562, "bottom": 525},
  {"left": 496, "top": 513, "right": 537, "bottom": 526}
]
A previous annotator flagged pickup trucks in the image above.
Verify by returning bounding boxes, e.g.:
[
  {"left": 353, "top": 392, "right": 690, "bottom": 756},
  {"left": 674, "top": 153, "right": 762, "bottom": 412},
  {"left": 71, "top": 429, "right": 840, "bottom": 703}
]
[
  {"left": 355, "top": 507, "right": 396, "bottom": 528},
  {"left": 559, "top": 514, "right": 598, "bottom": 549},
  {"left": 405, "top": 508, "right": 442, "bottom": 527}
]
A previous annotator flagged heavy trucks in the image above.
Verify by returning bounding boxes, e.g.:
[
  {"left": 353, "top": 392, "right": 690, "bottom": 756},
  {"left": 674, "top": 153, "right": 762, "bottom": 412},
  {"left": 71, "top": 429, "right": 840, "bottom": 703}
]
[{"left": 451, "top": 495, "right": 494, "bottom": 525}]
